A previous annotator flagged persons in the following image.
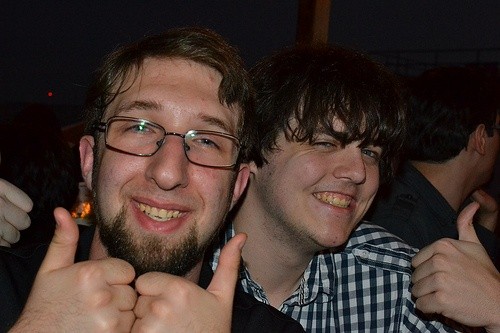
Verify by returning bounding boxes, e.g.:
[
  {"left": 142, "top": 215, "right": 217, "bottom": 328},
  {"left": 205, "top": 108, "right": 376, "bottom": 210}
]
[
  {"left": 0, "top": 21, "right": 305, "bottom": 332},
  {"left": 1, "top": 40, "right": 500, "bottom": 333},
  {"left": 365, "top": 63, "right": 500, "bottom": 263}
]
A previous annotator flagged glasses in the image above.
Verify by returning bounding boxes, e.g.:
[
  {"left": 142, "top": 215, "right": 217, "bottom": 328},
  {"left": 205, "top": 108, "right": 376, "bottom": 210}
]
[{"left": 95, "top": 115, "right": 243, "bottom": 167}]
